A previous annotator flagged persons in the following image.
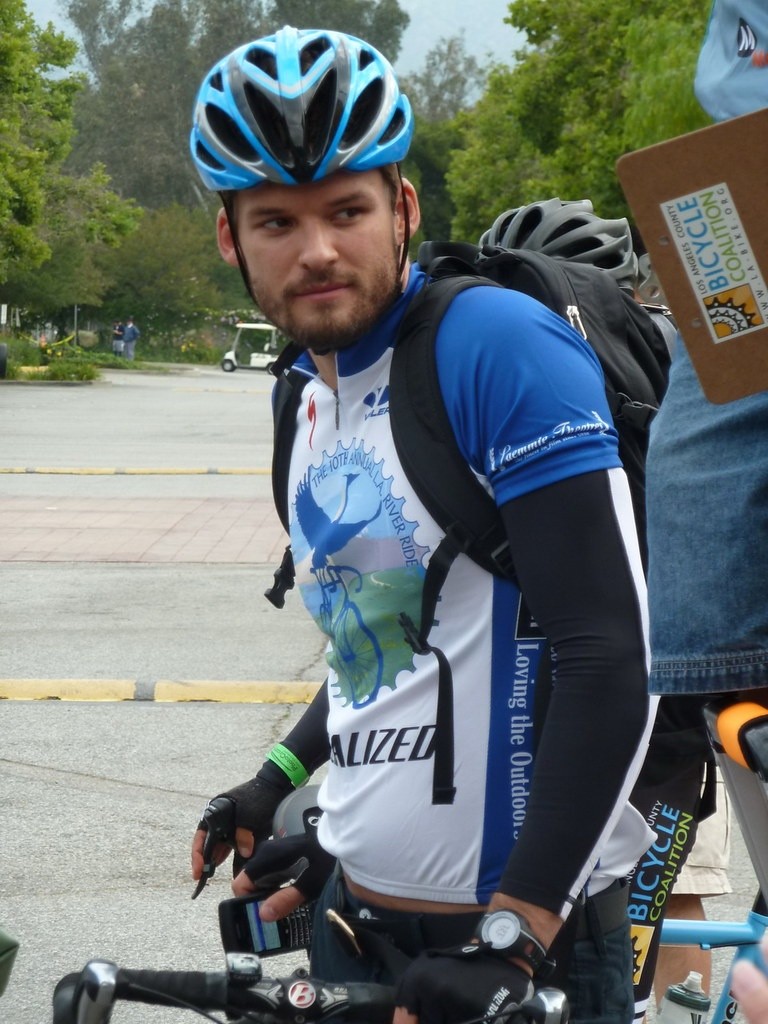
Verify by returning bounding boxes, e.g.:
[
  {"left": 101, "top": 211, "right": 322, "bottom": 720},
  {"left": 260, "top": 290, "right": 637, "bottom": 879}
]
[
  {"left": 188, "top": 23, "right": 664, "bottom": 1024},
  {"left": 476, "top": 0, "right": 767, "bottom": 1024},
  {"left": 111, "top": 315, "right": 140, "bottom": 360}
]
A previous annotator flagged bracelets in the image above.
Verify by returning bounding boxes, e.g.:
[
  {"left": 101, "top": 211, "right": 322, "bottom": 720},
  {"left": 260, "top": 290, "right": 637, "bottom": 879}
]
[{"left": 265, "top": 743, "right": 311, "bottom": 789}]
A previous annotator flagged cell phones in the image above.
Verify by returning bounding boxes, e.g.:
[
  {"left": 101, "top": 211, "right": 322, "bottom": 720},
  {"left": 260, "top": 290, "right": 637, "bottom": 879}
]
[{"left": 217, "top": 887, "right": 320, "bottom": 958}]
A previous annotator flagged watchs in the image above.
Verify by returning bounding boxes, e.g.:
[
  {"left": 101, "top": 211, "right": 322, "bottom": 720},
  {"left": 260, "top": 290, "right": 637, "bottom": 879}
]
[{"left": 468, "top": 908, "right": 560, "bottom": 978}]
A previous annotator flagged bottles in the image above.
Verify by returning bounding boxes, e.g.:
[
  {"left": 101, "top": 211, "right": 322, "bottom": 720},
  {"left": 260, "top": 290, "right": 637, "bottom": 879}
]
[{"left": 652, "top": 970, "right": 711, "bottom": 1024}]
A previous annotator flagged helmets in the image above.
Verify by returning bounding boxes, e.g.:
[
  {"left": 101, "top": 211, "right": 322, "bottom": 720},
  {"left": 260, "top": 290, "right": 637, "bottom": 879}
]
[
  {"left": 188, "top": 24, "right": 415, "bottom": 191},
  {"left": 476, "top": 195, "right": 639, "bottom": 300}
]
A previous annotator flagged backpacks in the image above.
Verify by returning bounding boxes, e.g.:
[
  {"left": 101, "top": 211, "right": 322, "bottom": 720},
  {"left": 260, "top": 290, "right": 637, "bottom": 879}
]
[{"left": 388, "top": 240, "right": 671, "bottom": 588}]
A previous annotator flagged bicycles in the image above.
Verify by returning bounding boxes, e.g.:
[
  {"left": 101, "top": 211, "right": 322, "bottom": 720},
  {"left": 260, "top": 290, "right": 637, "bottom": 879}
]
[{"left": 52, "top": 696, "right": 768, "bottom": 1024}]
